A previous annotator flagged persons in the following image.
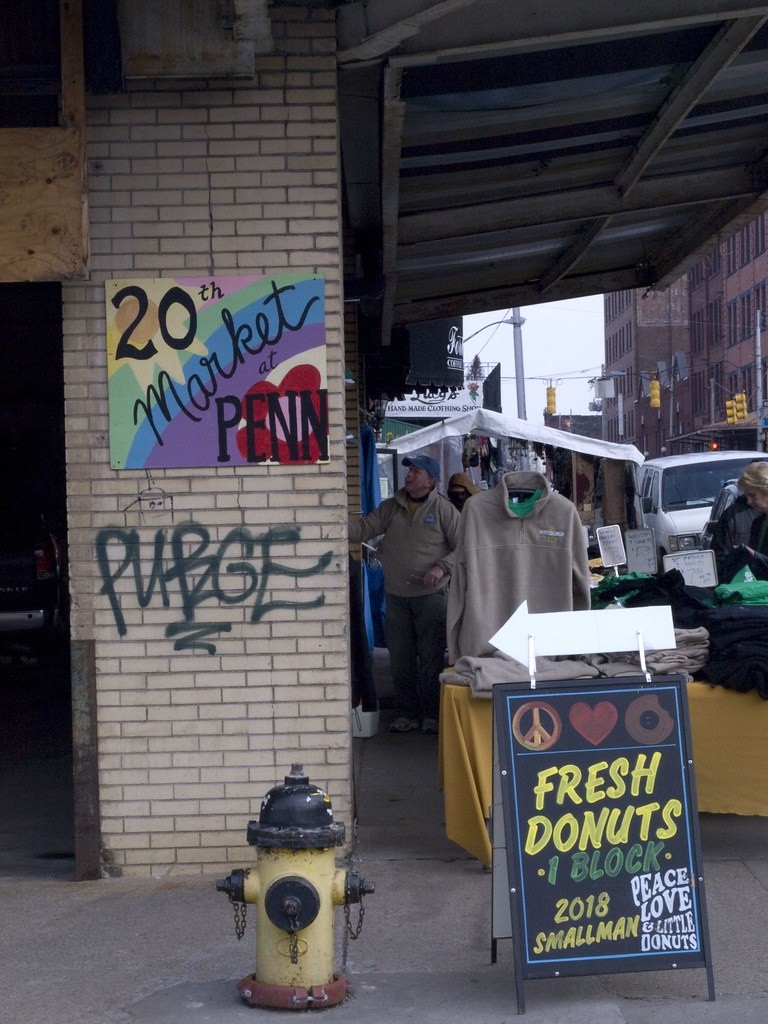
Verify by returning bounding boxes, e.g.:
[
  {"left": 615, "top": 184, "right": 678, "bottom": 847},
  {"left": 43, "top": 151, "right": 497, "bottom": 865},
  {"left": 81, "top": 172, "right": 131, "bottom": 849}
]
[
  {"left": 731, "top": 461, "right": 768, "bottom": 583},
  {"left": 447, "top": 473, "right": 480, "bottom": 515},
  {"left": 710, "top": 495, "right": 762, "bottom": 569},
  {"left": 345, "top": 453, "right": 466, "bottom": 734}
]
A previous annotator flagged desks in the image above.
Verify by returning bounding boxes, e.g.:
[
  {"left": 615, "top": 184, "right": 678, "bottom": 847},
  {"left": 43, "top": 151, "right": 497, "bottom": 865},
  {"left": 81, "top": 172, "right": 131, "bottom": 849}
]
[{"left": 440, "top": 667, "right": 768, "bottom": 875}]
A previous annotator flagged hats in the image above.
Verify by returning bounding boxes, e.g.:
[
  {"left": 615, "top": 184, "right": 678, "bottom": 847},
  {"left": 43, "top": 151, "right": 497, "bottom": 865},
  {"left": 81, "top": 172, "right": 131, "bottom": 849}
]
[{"left": 402, "top": 455, "right": 440, "bottom": 478}]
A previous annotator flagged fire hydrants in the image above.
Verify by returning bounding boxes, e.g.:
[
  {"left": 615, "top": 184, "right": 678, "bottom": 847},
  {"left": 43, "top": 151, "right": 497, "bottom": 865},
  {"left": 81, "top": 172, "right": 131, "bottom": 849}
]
[{"left": 216, "top": 762, "right": 375, "bottom": 1010}]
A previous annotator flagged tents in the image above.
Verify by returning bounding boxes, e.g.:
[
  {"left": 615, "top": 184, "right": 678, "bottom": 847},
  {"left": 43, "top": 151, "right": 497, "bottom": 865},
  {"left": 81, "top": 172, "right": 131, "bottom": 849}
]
[{"left": 374, "top": 408, "right": 653, "bottom": 531}]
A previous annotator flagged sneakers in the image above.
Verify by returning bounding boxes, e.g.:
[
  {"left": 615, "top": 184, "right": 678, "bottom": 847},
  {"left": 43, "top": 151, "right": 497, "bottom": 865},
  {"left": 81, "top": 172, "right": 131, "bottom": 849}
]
[
  {"left": 422, "top": 719, "right": 438, "bottom": 733},
  {"left": 389, "top": 717, "right": 419, "bottom": 731}
]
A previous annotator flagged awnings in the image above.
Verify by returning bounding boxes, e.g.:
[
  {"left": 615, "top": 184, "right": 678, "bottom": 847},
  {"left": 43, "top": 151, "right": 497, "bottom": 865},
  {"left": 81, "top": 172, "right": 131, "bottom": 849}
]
[{"left": 665, "top": 424, "right": 768, "bottom": 450}]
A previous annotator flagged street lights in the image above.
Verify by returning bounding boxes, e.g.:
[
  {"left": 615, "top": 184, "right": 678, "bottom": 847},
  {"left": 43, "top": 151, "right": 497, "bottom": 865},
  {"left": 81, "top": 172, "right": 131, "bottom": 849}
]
[{"left": 608, "top": 354, "right": 674, "bottom": 437}]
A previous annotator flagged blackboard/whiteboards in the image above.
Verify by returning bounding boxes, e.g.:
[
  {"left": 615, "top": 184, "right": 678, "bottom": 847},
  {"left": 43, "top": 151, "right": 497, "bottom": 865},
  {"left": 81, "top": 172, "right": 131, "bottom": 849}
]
[{"left": 493, "top": 675, "right": 711, "bottom": 979}]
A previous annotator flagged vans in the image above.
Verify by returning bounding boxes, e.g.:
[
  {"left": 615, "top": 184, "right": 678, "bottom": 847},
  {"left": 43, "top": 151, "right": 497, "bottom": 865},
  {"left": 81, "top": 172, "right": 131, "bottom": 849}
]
[
  {"left": 0, "top": 510, "right": 62, "bottom": 632},
  {"left": 638, "top": 451, "right": 768, "bottom": 577}
]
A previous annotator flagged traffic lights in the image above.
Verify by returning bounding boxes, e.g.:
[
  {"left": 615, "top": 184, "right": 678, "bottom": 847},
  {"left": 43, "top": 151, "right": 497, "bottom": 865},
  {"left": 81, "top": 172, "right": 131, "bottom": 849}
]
[
  {"left": 713, "top": 443, "right": 717, "bottom": 448},
  {"left": 736, "top": 394, "right": 747, "bottom": 420},
  {"left": 725, "top": 400, "right": 737, "bottom": 425}
]
